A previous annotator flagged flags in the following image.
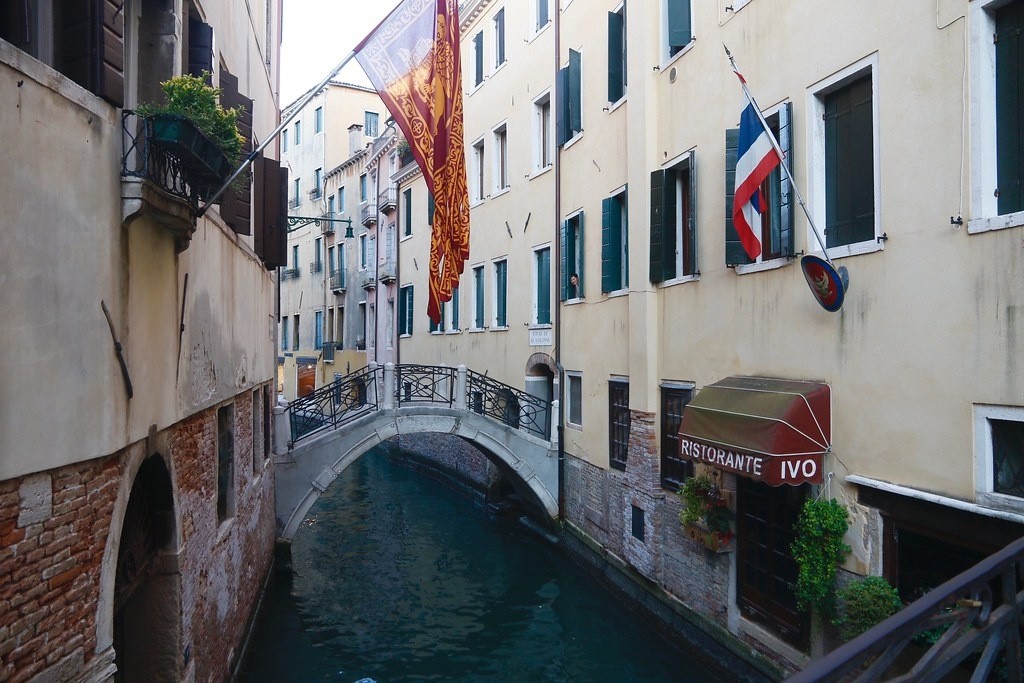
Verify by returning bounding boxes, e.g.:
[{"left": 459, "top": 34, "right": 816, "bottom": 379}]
[
  {"left": 732, "top": 72, "right": 786, "bottom": 261},
  {"left": 353, "top": 1, "right": 471, "bottom": 324}
]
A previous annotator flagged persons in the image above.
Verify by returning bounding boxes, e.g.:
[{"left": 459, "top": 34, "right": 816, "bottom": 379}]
[{"left": 569, "top": 273, "right": 579, "bottom": 295}]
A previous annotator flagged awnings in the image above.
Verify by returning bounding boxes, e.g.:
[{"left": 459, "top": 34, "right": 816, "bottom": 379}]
[{"left": 678, "top": 374, "right": 831, "bottom": 486}]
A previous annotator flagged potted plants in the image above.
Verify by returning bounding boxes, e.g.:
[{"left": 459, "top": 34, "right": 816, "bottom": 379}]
[
  {"left": 787, "top": 498, "right": 852, "bottom": 614},
  {"left": 134, "top": 68, "right": 255, "bottom": 194},
  {"left": 678, "top": 501, "right": 733, "bottom": 554},
  {"left": 396, "top": 140, "right": 414, "bottom": 167},
  {"left": 678, "top": 475, "right": 711, "bottom": 499}
]
